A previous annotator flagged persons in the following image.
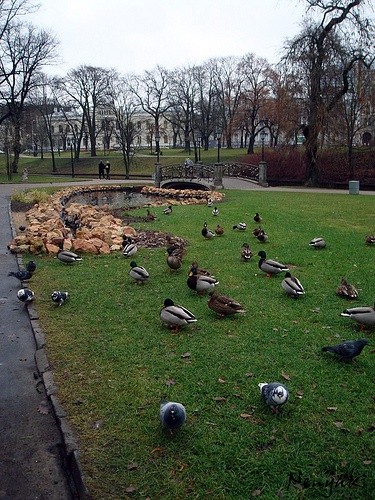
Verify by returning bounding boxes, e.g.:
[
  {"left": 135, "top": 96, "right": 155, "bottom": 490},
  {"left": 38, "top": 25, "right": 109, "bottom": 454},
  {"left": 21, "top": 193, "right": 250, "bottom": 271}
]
[
  {"left": 185, "top": 157, "right": 194, "bottom": 179},
  {"left": 98, "top": 159, "right": 110, "bottom": 180}
]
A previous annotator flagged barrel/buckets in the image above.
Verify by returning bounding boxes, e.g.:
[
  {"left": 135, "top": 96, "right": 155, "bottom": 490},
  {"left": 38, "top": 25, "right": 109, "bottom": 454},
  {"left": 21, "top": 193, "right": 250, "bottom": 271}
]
[{"left": 349, "top": 181, "right": 359, "bottom": 195}]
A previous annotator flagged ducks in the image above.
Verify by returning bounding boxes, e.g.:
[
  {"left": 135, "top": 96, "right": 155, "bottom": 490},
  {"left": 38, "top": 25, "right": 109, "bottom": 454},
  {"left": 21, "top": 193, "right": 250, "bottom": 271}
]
[
  {"left": 306, "top": 237, "right": 327, "bottom": 248},
  {"left": 339, "top": 307, "right": 375, "bottom": 331},
  {"left": 55, "top": 248, "right": 83, "bottom": 264},
  {"left": 130, "top": 261, "right": 151, "bottom": 283},
  {"left": 254, "top": 250, "right": 289, "bottom": 277},
  {"left": 158, "top": 298, "right": 199, "bottom": 333},
  {"left": 281, "top": 270, "right": 306, "bottom": 298}
]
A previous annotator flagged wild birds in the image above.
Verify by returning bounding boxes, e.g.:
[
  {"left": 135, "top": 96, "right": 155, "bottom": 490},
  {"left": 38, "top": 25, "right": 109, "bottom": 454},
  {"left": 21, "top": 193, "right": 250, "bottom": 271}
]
[
  {"left": 321, "top": 337, "right": 370, "bottom": 364},
  {"left": 19, "top": 204, "right": 375, "bottom": 272},
  {"left": 165, "top": 247, "right": 182, "bottom": 273},
  {"left": 205, "top": 291, "right": 246, "bottom": 318},
  {"left": 335, "top": 278, "right": 358, "bottom": 298},
  {"left": 158, "top": 398, "right": 187, "bottom": 435},
  {"left": 257, "top": 382, "right": 290, "bottom": 413},
  {"left": 187, "top": 268, "right": 219, "bottom": 297},
  {"left": 50, "top": 290, "right": 69, "bottom": 306},
  {"left": 8, "top": 267, "right": 34, "bottom": 285},
  {"left": 16, "top": 288, "right": 36, "bottom": 302},
  {"left": 241, "top": 243, "right": 253, "bottom": 262}
]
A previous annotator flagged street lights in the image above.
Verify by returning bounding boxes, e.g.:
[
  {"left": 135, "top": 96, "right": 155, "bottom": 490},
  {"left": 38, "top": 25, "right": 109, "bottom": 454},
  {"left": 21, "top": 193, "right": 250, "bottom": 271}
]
[
  {"left": 154, "top": 135, "right": 163, "bottom": 182},
  {"left": 214, "top": 129, "right": 224, "bottom": 186},
  {"left": 258, "top": 129, "right": 268, "bottom": 184}
]
